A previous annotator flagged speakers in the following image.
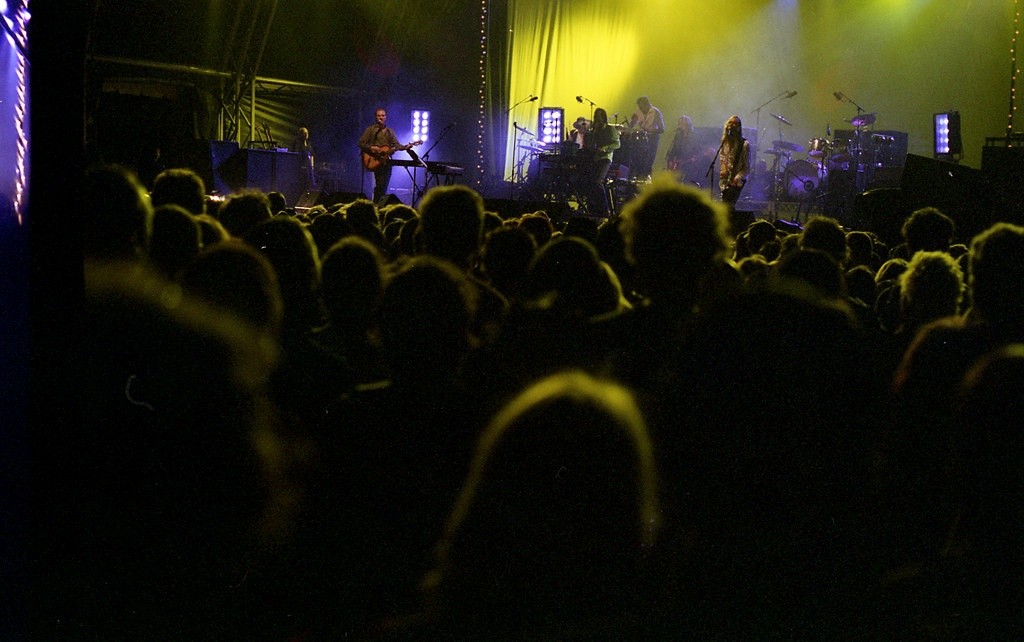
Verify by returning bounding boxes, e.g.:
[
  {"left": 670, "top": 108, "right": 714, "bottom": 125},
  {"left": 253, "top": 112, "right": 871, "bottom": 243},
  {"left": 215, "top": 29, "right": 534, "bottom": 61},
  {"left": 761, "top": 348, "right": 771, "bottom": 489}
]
[
  {"left": 728, "top": 209, "right": 756, "bottom": 238},
  {"left": 854, "top": 147, "right": 1024, "bottom": 250},
  {"left": 189, "top": 139, "right": 404, "bottom": 210}
]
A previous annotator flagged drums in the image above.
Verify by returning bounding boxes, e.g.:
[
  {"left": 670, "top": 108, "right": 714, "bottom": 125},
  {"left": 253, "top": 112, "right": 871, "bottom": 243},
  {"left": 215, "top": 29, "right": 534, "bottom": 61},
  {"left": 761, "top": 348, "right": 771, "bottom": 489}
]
[
  {"left": 841, "top": 148, "right": 866, "bottom": 173},
  {"left": 829, "top": 139, "right": 852, "bottom": 163},
  {"left": 782, "top": 159, "right": 829, "bottom": 201},
  {"left": 630, "top": 129, "right": 651, "bottom": 151},
  {"left": 808, "top": 136, "right": 828, "bottom": 158}
]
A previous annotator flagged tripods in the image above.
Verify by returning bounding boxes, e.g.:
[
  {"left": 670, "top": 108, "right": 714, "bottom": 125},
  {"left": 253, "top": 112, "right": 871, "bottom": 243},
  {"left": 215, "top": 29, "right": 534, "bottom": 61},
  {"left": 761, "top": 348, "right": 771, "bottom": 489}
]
[{"left": 505, "top": 151, "right": 533, "bottom": 184}]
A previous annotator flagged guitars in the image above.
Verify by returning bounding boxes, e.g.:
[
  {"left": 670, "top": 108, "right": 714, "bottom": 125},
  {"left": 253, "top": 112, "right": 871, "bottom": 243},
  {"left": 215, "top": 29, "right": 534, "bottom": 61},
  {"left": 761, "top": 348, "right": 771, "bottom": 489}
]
[
  {"left": 718, "top": 169, "right": 746, "bottom": 193},
  {"left": 256, "top": 122, "right": 277, "bottom": 149},
  {"left": 581, "top": 122, "right": 604, "bottom": 168},
  {"left": 363, "top": 140, "right": 423, "bottom": 171},
  {"left": 666, "top": 156, "right": 701, "bottom": 172}
]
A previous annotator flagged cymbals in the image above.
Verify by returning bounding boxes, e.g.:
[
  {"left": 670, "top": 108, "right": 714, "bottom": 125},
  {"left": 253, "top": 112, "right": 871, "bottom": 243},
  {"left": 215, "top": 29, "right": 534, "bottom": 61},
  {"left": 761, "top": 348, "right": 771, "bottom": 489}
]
[
  {"left": 516, "top": 126, "right": 533, "bottom": 135},
  {"left": 851, "top": 113, "right": 877, "bottom": 127},
  {"left": 608, "top": 123, "right": 625, "bottom": 131},
  {"left": 768, "top": 111, "right": 792, "bottom": 125},
  {"left": 573, "top": 119, "right": 593, "bottom": 130},
  {"left": 517, "top": 144, "right": 544, "bottom": 152},
  {"left": 771, "top": 140, "right": 805, "bottom": 152}
]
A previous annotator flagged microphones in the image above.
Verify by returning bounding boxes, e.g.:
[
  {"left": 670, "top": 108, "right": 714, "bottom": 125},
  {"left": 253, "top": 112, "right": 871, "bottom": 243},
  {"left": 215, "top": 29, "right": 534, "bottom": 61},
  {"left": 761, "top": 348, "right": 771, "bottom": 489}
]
[
  {"left": 728, "top": 127, "right": 733, "bottom": 135},
  {"left": 529, "top": 97, "right": 538, "bottom": 101},
  {"left": 833, "top": 93, "right": 844, "bottom": 102},
  {"left": 576, "top": 97, "right": 582, "bottom": 102},
  {"left": 827, "top": 124, "right": 831, "bottom": 136},
  {"left": 783, "top": 91, "right": 797, "bottom": 99},
  {"left": 444, "top": 122, "right": 456, "bottom": 131}
]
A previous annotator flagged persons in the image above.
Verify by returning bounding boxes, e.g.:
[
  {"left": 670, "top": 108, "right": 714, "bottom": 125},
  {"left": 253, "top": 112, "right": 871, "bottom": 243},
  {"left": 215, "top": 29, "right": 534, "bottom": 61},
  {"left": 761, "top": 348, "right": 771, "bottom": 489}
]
[
  {"left": 573, "top": 108, "right": 621, "bottom": 218},
  {"left": 667, "top": 115, "right": 750, "bottom": 211},
  {"left": 1, "top": 165, "right": 1023, "bottom": 641},
  {"left": 628, "top": 97, "right": 665, "bottom": 178},
  {"left": 292, "top": 128, "right": 310, "bottom": 190},
  {"left": 358, "top": 106, "right": 413, "bottom": 204}
]
